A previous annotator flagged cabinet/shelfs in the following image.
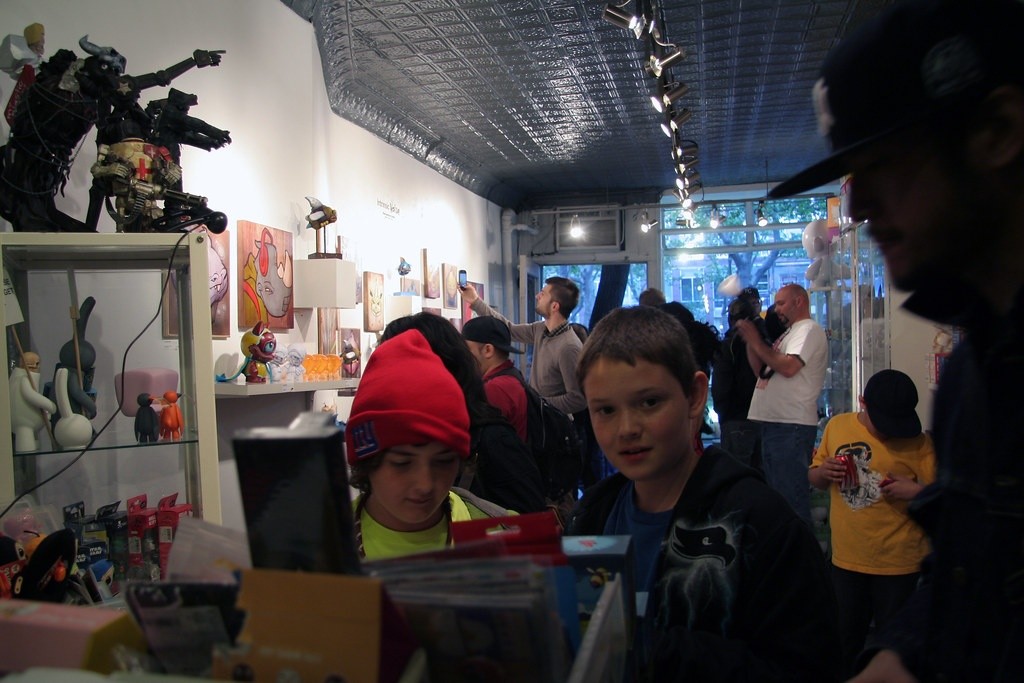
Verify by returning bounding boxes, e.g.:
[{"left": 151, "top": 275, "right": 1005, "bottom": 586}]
[{"left": 0, "top": 226, "right": 223, "bottom": 555}]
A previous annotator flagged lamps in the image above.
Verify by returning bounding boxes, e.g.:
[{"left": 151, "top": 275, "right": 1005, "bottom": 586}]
[
  {"left": 570, "top": 213, "right": 583, "bottom": 237},
  {"left": 603, "top": 0, "right": 770, "bottom": 232},
  {"left": 292, "top": 258, "right": 356, "bottom": 353}
]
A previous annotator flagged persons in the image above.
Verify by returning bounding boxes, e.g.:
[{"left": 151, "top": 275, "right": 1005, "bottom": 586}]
[
  {"left": 461, "top": 315, "right": 531, "bottom": 446},
  {"left": 346, "top": 330, "right": 525, "bottom": 562},
  {"left": 736, "top": 284, "right": 827, "bottom": 534},
  {"left": 808, "top": 370, "right": 938, "bottom": 683},
  {"left": 659, "top": 300, "right": 720, "bottom": 435},
  {"left": 379, "top": 312, "right": 551, "bottom": 514},
  {"left": 457, "top": 276, "right": 588, "bottom": 416},
  {"left": 639, "top": 288, "right": 665, "bottom": 310},
  {"left": 738, "top": 287, "right": 767, "bottom": 344},
  {"left": 560, "top": 307, "right": 831, "bottom": 683},
  {"left": 712, "top": 299, "right": 758, "bottom": 465},
  {"left": 766, "top": 303, "right": 786, "bottom": 346},
  {"left": 765, "top": 0, "right": 1024, "bottom": 683}
]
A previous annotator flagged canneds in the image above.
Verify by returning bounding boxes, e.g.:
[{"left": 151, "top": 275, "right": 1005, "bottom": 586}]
[{"left": 833, "top": 452, "right": 859, "bottom": 491}]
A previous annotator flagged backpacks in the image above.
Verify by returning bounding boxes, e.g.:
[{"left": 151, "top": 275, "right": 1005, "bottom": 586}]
[{"left": 483, "top": 367, "right": 580, "bottom": 501}]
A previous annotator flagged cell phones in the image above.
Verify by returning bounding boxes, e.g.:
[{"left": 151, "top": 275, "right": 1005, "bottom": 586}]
[{"left": 459, "top": 269, "right": 468, "bottom": 291}]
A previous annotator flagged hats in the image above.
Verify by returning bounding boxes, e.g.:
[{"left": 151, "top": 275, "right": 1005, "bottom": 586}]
[
  {"left": 765, "top": 0, "right": 1024, "bottom": 200},
  {"left": 461, "top": 315, "right": 525, "bottom": 354},
  {"left": 864, "top": 369, "right": 922, "bottom": 437},
  {"left": 344, "top": 329, "right": 469, "bottom": 464}
]
